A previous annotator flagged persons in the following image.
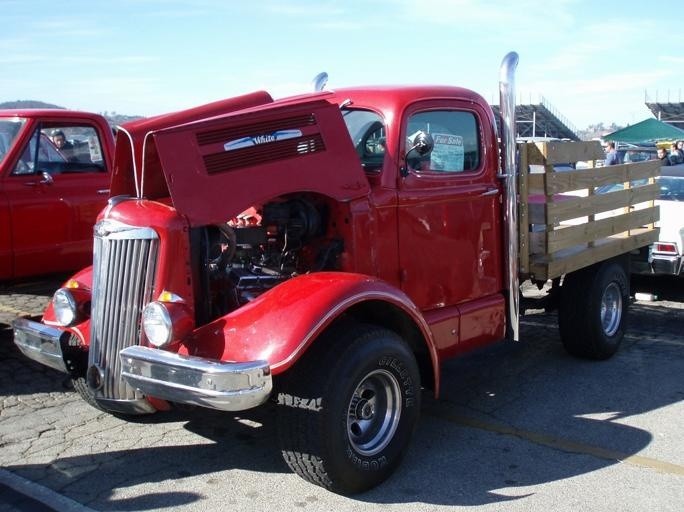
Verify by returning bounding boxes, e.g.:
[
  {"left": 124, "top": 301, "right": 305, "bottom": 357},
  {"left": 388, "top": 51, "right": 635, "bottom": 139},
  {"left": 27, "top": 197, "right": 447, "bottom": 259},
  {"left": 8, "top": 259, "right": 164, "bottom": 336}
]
[
  {"left": 603, "top": 137, "right": 683, "bottom": 167},
  {"left": 50, "top": 129, "right": 75, "bottom": 162}
]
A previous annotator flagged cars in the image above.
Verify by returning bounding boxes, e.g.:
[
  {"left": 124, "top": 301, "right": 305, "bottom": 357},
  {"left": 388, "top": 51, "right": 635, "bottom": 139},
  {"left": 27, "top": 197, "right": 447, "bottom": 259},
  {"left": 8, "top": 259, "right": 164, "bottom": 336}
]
[
  {"left": 604, "top": 141, "right": 658, "bottom": 163},
  {"left": 581, "top": 163, "right": 684, "bottom": 283}
]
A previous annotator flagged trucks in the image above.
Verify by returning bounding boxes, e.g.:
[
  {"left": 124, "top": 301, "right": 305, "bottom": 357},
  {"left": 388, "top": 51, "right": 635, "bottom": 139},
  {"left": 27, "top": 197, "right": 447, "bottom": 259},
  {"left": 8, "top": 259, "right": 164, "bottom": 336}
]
[
  {"left": 0, "top": 107, "right": 118, "bottom": 287},
  {"left": 11, "top": 50, "right": 667, "bottom": 493}
]
